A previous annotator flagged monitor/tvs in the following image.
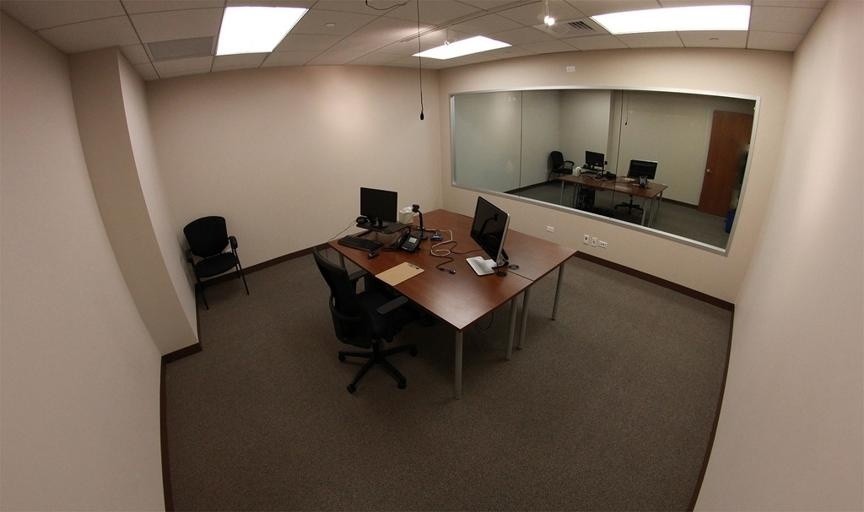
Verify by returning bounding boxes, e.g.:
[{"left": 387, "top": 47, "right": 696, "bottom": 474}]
[
  {"left": 584, "top": 151, "right": 605, "bottom": 169},
  {"left": 360, "top": 186, "right": 397, "bottom": 228},
  {"left": 466, "top": 196, "right": 509, "bottom": 276},
  {"left": 628, "top": 159, "right": 657, "bottom": 186}
]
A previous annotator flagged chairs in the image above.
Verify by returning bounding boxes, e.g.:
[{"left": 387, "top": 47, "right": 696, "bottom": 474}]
[
  {"left": 312, "top": 248, "right": 420, "bottom": 394},
  {"left": 548, "top": 150, "right": 574, "bottom": 188},
  {"left": 183, "top": 215, "right": 250, "bottom": 310}
]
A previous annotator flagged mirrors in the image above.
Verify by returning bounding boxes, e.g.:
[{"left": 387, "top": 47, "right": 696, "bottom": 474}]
[{"left": 449, "top": 81, "right": 761, "bottom": 255}]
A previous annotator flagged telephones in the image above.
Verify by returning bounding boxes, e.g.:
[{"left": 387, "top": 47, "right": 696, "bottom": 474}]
[{"left": 394, "top": 232, "right": 420, "bottom": 252}]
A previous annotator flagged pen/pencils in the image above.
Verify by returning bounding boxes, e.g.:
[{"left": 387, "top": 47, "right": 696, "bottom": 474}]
[{"left": 436, "top": 231, "right": 437, "bottom": 236}]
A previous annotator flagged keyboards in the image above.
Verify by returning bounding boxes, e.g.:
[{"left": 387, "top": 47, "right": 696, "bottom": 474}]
[{"left": 338, "top": 235, "right": 383, "bottom": 251}]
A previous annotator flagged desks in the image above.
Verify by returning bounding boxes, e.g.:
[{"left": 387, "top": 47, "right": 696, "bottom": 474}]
[
  {"left": 557, "top": 171, "right": 669, "bottom": 227},
  {"left": 411, "top": 209, "right": 580, "bottom": 350},
  {"left": 327, "top": 228, "right": 533, "bottom": 402}
]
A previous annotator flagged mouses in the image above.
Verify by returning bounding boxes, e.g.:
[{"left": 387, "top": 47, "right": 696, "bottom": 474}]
[{"left": 367, "top": 252, "right": 378, "bottom": 258}]
